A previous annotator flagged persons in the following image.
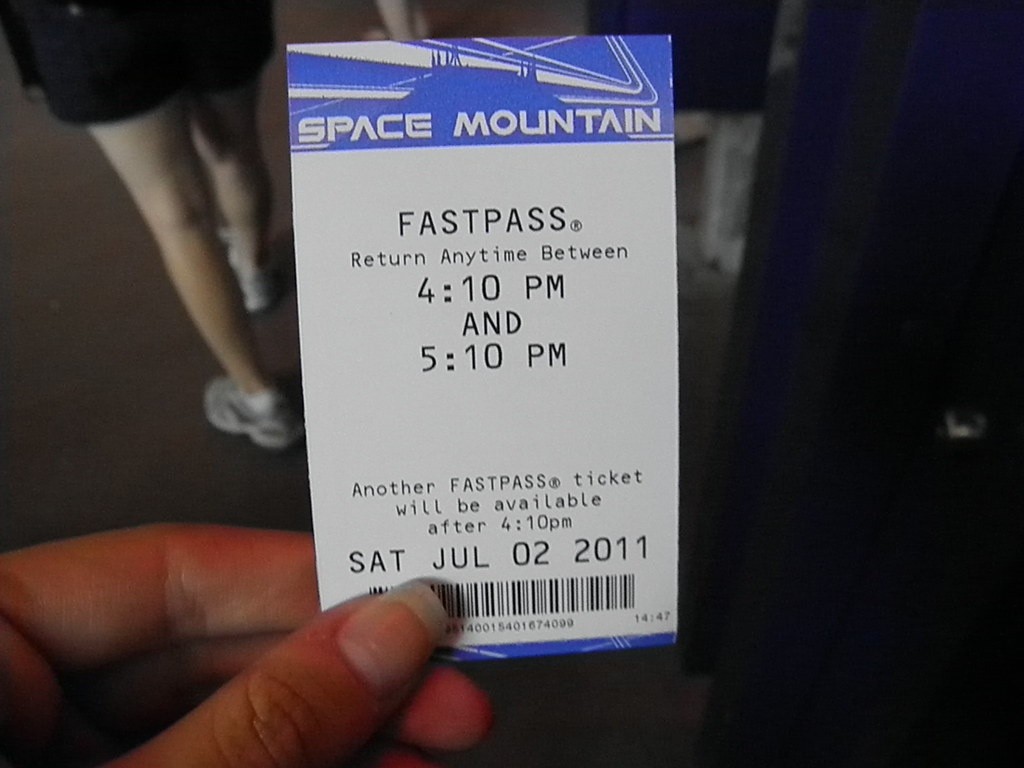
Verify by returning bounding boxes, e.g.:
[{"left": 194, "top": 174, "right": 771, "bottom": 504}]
[
  {"left": 359, "top": 0, "right": 433, "bottom": 41},
  {"left": 1, "top": 516, "right": 499, "bottom": 768},
  {"left": 1, "top": 0, "right": 306, "bottom": 455}
]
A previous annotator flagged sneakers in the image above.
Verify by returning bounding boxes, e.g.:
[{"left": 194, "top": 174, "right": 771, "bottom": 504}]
[
  {"left": 239, "top": 272, "right": 282, "bottom": 313},
  {"left": 203, "top": 374, "right": 305, "bottom": 451}
]
